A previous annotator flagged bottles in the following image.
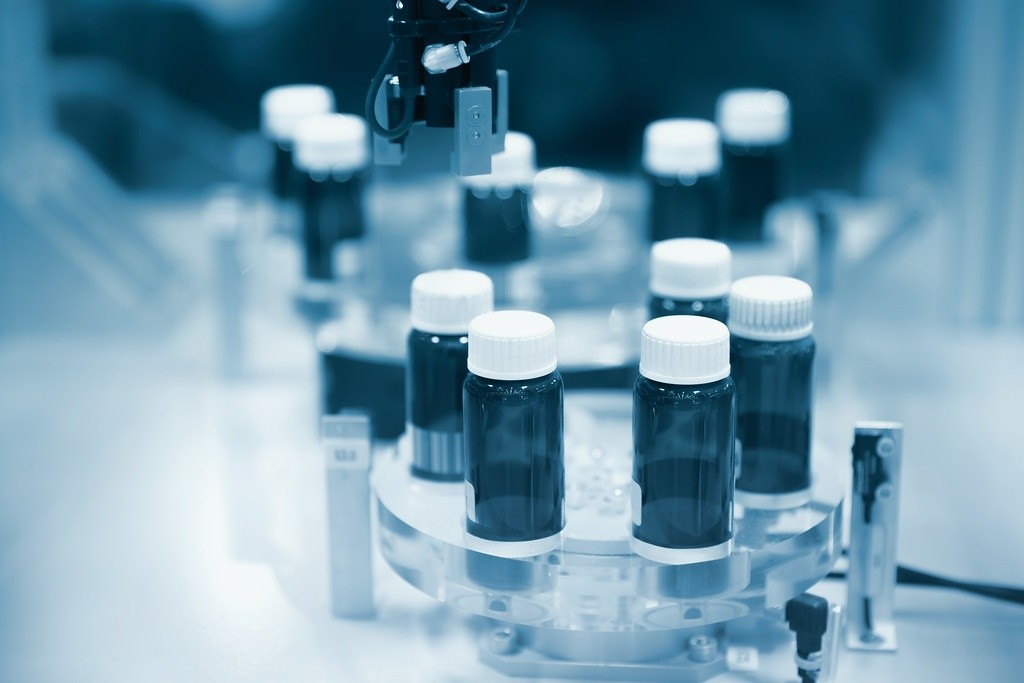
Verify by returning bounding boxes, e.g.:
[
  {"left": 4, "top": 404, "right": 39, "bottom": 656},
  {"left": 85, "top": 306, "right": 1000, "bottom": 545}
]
[
  {"left": 643, "top": 118, "right": 721, "bottom": 243},
  {"left": 715, "top": 87, "right": 791, "bottom": 248},
  {"left": 298, "top": 115, "right": 369, "bottom": 283},
  {"left": 726, "top": 277, "right": 816, "bottom": 511},
  {"left": 459, "top": 131, "right": 535, "bottom": 265},
  {"left": 261, "top": 84, "right": 334, "bottom": 239},
  {"left": 464, "top": 310, "right": 563, "bottom": 557},
  {"left": 403, "top": 268, "right": 493, "bottom": 500},
  {"left": 648, "top": 236, "right": 733, "bottom": 323},
  {"left": 632, "top": 315, "right": 734, "bottom": 565}
]
